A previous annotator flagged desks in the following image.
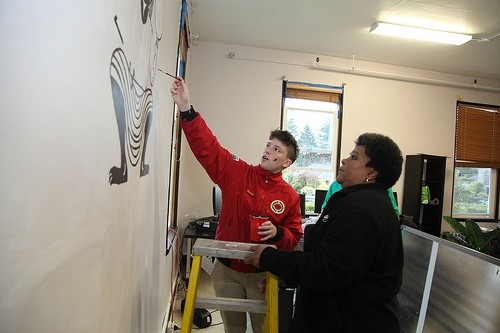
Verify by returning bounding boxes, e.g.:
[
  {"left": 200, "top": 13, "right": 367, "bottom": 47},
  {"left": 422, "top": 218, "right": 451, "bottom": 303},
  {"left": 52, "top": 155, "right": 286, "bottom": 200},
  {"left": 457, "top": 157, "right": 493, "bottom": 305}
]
[{"left": 183, "top": 224, "right": 216, "bottom": 279}]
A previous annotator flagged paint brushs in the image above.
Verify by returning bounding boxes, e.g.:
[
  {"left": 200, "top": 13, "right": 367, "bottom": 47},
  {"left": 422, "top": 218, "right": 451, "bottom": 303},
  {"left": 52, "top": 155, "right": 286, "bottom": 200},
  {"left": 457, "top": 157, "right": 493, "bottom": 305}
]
[{"left": 157, "top": 68, "right": 185, "bottom": 83}]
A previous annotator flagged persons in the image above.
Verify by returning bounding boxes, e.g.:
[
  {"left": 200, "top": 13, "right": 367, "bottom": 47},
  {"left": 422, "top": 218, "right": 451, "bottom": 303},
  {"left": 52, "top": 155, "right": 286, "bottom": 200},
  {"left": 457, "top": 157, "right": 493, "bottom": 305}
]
[
  {"left": 245, "top": 132, "right": 406, "bottom": 333},
  {"left": 171, "top": 78, "right": 302, "bottom": 333}
]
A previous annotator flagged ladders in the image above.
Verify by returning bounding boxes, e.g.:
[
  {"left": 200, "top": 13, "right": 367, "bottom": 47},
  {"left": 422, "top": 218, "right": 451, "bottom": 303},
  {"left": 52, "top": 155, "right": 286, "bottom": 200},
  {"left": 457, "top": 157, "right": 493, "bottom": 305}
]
[{"left": 180, "top": 237, "right": 282, "bottom": 333}]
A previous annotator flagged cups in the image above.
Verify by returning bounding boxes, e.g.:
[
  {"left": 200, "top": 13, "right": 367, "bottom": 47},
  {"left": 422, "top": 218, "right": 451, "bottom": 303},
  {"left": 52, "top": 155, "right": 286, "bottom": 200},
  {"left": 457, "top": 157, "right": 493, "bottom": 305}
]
[{"left": 249, "top": 214, "right": 269, "bottom": 242}]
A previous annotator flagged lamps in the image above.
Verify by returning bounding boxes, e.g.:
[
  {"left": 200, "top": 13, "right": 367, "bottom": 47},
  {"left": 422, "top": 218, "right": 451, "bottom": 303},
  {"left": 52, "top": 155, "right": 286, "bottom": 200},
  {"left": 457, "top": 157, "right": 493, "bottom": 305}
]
[{"left": 370, "top": 22, "right": 472, "bottom": 46}]
